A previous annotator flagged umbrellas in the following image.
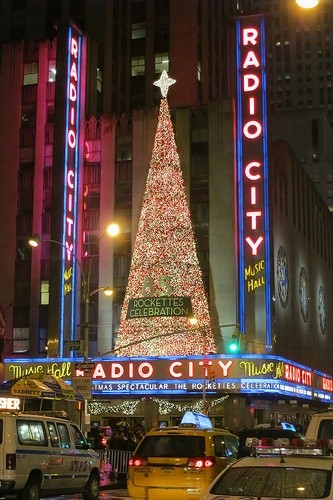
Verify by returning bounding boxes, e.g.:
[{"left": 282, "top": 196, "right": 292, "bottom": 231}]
[{"left": 0, "top": 372, "right": 86, "bottom": 411}]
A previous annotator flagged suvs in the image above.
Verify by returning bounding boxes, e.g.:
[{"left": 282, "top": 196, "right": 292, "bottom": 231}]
[{"left": 125, "top": 412, "right": 242, "bottom": 500}]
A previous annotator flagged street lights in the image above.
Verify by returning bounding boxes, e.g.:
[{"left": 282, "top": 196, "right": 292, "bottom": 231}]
[{"left": 29, "top": 223, "right": 119, "bottom": 431}]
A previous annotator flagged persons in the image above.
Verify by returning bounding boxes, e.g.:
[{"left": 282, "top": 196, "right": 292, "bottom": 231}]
[{"left": 91, "top": 426, "right": 155, "bottom": 481}]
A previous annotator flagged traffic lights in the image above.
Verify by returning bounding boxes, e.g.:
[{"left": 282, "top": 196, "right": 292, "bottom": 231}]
[{"left": 228, "top": 333, "right": 244, "bottom": 353}]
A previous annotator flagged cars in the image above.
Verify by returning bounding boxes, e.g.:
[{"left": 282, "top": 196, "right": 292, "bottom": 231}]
[{"left": 203, "top": 436, "right": 333, "bottom": 500}]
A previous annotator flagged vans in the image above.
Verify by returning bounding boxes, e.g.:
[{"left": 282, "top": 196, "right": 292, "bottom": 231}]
[
  {"left": 0, "top": 398, "right": 101, "bottom": 500},
  {"left": 300, "top": 409, "right": 333, "bottom": 450}
]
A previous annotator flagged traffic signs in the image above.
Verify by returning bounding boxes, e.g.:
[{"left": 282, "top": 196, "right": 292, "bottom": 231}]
[{"left": 75, "top": 362, "right": 95, "bottom": 371}]
